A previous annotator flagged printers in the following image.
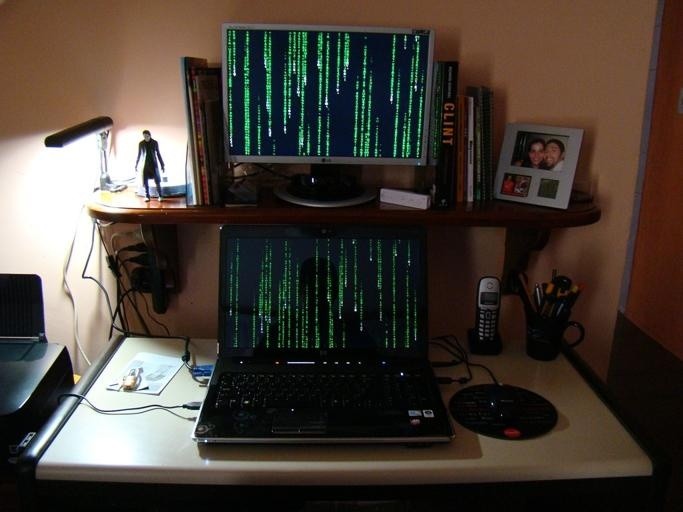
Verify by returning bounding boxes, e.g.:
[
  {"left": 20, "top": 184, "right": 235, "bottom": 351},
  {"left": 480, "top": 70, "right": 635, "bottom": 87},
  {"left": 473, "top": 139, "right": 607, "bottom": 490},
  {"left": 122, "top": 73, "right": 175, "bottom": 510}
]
[{"left": 0, "top": 274, "right": 74, "bottom": 474}]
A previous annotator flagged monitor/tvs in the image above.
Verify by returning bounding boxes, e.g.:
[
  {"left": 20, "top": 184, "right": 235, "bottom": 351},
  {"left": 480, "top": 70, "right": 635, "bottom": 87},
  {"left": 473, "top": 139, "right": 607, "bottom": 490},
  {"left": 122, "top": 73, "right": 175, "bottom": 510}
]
[{"left": 219, "top": 22, "right": 435, "bottom": 209}]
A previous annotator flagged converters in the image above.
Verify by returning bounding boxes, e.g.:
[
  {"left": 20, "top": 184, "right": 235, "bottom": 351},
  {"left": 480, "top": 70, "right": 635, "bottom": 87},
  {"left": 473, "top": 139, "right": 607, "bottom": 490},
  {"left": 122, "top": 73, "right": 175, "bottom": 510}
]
[{"left": 131, "top": 268, "right": 152, "bottom": 294}]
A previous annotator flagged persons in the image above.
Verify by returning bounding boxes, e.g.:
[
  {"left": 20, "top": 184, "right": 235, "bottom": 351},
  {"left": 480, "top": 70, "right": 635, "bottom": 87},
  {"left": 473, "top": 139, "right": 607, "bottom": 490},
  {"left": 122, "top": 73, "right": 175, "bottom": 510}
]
[
  {"left": 515, "top": 138, "right": 566, "bottom": 173},
  {"left": 134, "top": 130, "right": 165, "bottom": 202}
]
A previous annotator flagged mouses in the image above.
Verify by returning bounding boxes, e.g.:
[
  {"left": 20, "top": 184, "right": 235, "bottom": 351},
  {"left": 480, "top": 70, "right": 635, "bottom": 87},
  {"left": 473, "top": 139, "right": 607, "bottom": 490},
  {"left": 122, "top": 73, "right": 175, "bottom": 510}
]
[{"left": 486, "top": 383, "right": 517, "bottom": 418}]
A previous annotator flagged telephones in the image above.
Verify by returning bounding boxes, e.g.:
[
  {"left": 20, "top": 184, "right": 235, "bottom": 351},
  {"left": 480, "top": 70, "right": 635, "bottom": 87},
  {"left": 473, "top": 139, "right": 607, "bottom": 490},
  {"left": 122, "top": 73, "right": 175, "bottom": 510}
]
[{"left": 466, "top": 276, "right": 503, "bottom": 356}]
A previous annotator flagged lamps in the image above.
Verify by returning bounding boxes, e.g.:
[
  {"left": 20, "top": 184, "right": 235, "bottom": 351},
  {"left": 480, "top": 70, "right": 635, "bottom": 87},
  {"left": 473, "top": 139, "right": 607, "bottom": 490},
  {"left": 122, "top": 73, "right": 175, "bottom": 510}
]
[{"left": 45, "top": 116, "right": 127, "bottom": 192}]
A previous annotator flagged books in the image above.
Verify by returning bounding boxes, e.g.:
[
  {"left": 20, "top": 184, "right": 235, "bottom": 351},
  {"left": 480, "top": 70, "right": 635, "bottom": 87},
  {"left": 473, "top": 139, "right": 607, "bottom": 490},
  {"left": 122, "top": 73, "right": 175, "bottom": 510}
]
[
  {"left": 433, "top": 60, "right": 495, "bottom": 206},
  {"left": 178, "top": 55, "right": 220, "bottom": 205}
]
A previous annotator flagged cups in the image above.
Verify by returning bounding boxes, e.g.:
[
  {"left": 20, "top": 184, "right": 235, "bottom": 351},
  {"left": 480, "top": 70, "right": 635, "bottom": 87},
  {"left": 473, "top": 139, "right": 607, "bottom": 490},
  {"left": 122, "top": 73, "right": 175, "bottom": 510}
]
[{"left": 523, "top": 301, "right": 586, "bottom": 361}]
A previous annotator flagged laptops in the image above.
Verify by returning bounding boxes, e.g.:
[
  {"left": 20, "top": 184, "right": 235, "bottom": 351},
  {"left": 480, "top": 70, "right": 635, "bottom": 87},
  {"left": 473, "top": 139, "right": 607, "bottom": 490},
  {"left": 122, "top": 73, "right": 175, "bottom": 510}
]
[{"left": 193, "top": 222, "right": 456, "bottom": 446}]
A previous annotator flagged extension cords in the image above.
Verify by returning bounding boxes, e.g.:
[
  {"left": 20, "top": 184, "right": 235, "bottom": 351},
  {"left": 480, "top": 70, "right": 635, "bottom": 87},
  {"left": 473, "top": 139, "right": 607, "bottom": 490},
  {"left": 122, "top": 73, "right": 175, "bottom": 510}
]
[{"left": 139, "top": 249, "right": 169, "bottom": 314}]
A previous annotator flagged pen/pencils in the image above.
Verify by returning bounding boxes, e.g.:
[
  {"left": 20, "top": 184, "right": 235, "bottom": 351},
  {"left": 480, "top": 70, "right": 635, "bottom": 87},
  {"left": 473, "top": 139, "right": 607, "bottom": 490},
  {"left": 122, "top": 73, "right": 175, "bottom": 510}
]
[{"left": 518, "top": 264, "right": 585, "bottom": 321}]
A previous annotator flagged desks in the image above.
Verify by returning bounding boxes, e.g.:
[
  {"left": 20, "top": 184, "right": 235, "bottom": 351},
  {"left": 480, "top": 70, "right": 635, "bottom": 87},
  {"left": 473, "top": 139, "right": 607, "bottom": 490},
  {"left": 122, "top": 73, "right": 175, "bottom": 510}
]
[{"left": 20, "top": 334, "right": 654, "bottom": 512}]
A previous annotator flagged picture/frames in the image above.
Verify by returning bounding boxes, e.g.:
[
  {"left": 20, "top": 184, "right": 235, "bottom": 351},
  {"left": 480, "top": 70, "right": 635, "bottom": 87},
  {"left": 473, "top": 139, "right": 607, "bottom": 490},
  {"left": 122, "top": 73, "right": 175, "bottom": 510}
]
[{"left": 493, "top": 123, "right": 586, "bottom": 210}]
